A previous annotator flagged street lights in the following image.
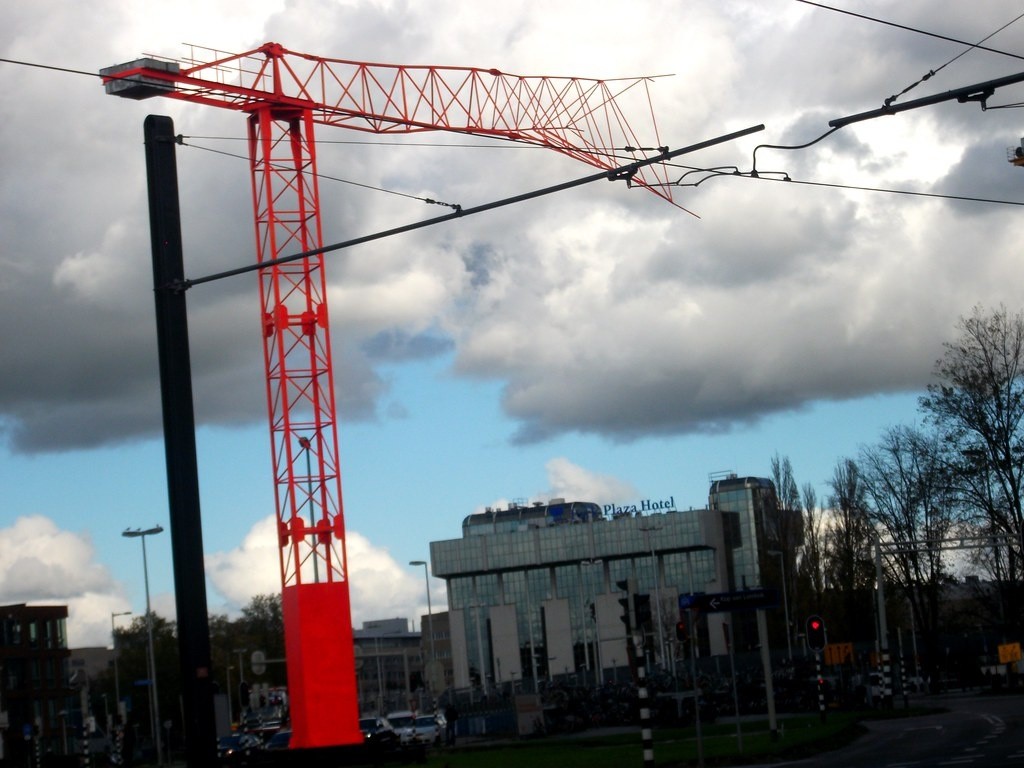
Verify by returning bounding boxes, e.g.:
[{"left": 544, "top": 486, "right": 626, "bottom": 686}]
[
  {"left": 408, "top": 560, "right": 445, "bottom": 710},
  {"left": 59, "top": 710, "right": 69, "bottom": 756},
  {"left": 111, "top": 611, "right": 132, "bottom": 726},
  {"left": 230, "top": 646, "right": 249, "bottom": 684},
  {"left": 580, "top": 555, "right": 606, "bottom": 689},
  {"left": 123, "top": 519, "right": 185, "bottom": 768}
]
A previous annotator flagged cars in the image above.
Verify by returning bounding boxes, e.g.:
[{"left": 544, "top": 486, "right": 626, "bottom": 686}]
[
  {"left": 216, "top": 685, "right": 416, "bottom": 758},
  {"left": 399, "top": 713, "right": 449, "bottom": 746}
]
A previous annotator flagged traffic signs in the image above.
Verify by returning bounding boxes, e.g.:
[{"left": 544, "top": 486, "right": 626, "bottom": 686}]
[{"left": 699, "top": 586, "right": 768, "bottom": 615}]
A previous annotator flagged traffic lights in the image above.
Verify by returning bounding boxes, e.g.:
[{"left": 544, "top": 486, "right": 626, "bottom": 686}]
[
  {"left": 806, "top": 619, "right": 829, "bottom": 653},
  {"left": 675, "top": 620, "right": 690, "bottom": 643},
  {"left": 617, "top": 590, "right": 653, "bottom": 647}
]
